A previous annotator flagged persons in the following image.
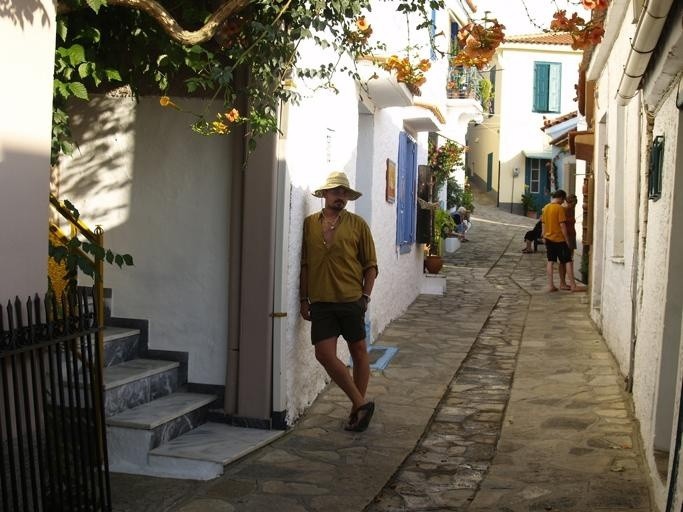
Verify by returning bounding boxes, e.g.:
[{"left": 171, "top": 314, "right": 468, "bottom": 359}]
[
  {"left": 300, "top": 171, "right": 379, "bottom": 432},
  {"left": 521, "top": 213, "right": 543, "bottom": 254},
  {"left": 558, "top": 194, "right": 578, "bottom": 290},
  {"left": 542, "top": 189, "right": 588, "bottom": 292},
  {"left": 449, "top": 205, "right": 469, "bottom": 243}
]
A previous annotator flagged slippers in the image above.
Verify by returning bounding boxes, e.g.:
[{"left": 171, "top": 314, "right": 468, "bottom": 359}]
[{"left": 344, "top": 402, "right": 374, "bottom": 431}]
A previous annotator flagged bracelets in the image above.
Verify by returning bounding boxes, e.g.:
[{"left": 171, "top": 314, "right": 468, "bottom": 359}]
[
  {"left": 300, "top": 295, "right": 308, "bottom": 300},
  {"left": 363, "top": 292, "right": 371, "bottom": 304}
]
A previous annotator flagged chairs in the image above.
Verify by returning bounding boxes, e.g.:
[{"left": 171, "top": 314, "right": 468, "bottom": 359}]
[{"left": 533, "top": 224, "right": 545, "bottom": 252}]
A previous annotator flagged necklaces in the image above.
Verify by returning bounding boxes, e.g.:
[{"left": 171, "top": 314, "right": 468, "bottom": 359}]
[{"left": 323, "top": 212, "right": 336, "bottom": 233}]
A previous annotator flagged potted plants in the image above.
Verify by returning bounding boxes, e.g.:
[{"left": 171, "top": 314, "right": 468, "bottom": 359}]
[
  {"left": 520, "top": 191, "right": 540, "bottom": 219},
  {"left": 424, "top": 238, "right": 444, "bottom": 275}
]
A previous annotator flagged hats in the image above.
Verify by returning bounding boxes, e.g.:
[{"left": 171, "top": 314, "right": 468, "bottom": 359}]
[{"left": 312, "top": 172, "right": 362, "bottom": 200}]
